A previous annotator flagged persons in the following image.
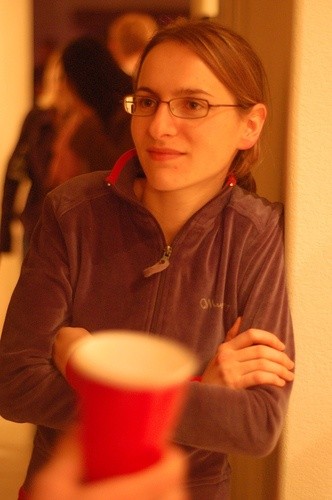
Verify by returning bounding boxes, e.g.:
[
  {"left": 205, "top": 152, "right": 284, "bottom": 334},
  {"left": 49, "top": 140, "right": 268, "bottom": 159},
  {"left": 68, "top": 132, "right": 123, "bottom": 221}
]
[
  {"left": 0, "top": 22, "right": 296, "bottom": 500},
  {"left": 26, "top": 425, "right": 189, "bottom": 500},
  {"left": 0, "top": 15, "right": 157, "bottom": 253}
]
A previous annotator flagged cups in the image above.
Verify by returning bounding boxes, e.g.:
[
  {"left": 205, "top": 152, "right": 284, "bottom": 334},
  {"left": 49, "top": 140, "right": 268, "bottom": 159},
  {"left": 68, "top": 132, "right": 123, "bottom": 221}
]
[{"left": 64, "top": 331, "right": 200, "bottom": 485}]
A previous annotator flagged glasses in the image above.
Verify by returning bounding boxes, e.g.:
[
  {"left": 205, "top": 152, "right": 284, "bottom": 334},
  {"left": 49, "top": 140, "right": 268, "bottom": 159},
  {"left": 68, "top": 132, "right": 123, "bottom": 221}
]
[{"left": 120, "top": 93, "right": 250, "bottom": 121}]
